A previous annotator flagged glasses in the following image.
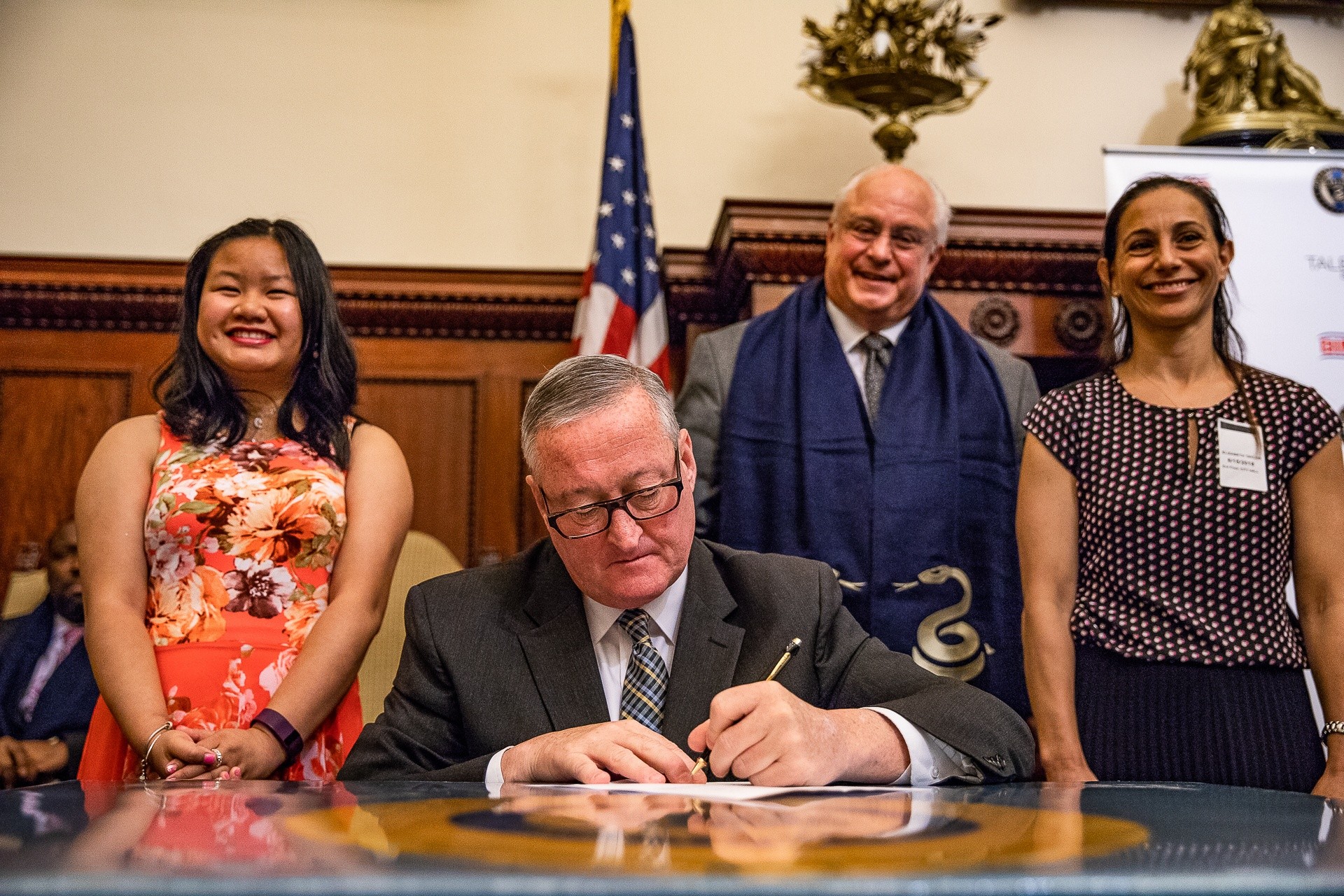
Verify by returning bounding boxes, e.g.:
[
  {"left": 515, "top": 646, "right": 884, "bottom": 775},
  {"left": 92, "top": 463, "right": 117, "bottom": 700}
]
[{"left": 538, "top": 438, "right": 684, "bottom": 539}]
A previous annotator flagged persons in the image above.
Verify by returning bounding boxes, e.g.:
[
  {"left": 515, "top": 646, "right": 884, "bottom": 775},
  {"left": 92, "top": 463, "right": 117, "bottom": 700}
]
[
  {"left": 329, "top": 353, "right": 1045, "bottom": 797},
  {"left": 76, "top": 218, "right": 416, "bottom": 816},
  {"left": 0, "top": 515, "right": 103, "bottom": 792},
  {"left": 671, "top": 161, "right": 1042, "bottom": 733},
  {"left": 1017, "top": 174, "right": 1344, "bottom": 803}
]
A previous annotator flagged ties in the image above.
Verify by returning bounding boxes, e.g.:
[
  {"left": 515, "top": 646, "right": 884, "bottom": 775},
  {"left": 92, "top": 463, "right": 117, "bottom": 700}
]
[
  {"left": 858, "top": 332, "right": 890, "bottom": 426},
  {"left": 618, "top": 608, "right": 669, "bottom": 735},
  {"left": 17, "top": 613, "right": 84, "bottom": 722}
]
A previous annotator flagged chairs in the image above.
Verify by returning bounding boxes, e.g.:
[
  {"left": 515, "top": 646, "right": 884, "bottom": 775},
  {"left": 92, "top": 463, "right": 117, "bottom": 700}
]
[{"left": 357, "top": 531, "right": 462, "bottom": 720}]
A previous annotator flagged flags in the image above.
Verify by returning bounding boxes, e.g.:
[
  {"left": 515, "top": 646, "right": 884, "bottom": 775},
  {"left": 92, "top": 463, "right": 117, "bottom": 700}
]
[{"left": 571, "top": 0, "right": 675, "bottom": 403}]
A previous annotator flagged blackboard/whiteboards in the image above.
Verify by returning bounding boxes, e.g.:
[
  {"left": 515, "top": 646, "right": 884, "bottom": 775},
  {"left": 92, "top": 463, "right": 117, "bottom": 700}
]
[{"left": 1104, "top": 145, "right": 1344, "bottom": 762}]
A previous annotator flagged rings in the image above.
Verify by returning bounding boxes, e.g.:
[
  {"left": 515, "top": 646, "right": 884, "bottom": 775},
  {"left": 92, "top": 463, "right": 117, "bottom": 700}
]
[{"left": 211, "top": 745, "right": 224, "bottom": 768}]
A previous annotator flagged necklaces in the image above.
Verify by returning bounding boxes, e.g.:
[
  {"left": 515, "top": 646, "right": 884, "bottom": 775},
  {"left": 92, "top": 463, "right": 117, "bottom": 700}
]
[{"left": 246, "top": 408, "right": 275, "bottom": 443}]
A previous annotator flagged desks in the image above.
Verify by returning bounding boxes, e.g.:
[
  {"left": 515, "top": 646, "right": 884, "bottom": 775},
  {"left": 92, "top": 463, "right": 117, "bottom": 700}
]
[{"left": 0, "top": 781, "right": 1344, "bottom": 896}]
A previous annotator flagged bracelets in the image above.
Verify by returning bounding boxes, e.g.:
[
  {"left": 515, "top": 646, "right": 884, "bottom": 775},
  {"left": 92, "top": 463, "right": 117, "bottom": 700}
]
[
  {"left": 248, "top": 705, "right": 303, "bottom": 760},
  {"left": 44, "top": 735, "right": 61, "bottom": 766},
  {"left": 136, "top": 722, "right": 177, "bottom": 782},
  {"left": 1321, "top": 719, "right": 1344, "bottom": 747}
]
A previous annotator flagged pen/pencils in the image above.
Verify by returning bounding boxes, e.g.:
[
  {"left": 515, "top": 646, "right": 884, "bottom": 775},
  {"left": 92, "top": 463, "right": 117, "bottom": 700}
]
[
  {"left": 693, "top": 802, "right": 710, "bottom": 820},
  {"left": 691, "top": 638, "right": 802, "bottom": 775}
]
